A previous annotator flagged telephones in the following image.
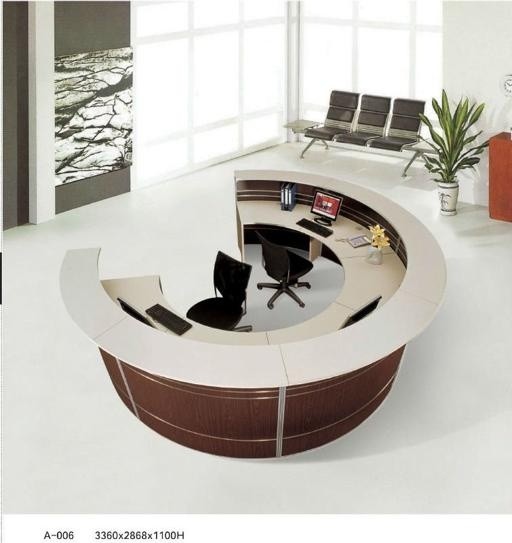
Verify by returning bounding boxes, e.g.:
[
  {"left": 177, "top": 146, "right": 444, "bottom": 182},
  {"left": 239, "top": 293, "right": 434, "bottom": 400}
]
[{"left": 347, "top": 233, "right": 371, "bottom": 248}]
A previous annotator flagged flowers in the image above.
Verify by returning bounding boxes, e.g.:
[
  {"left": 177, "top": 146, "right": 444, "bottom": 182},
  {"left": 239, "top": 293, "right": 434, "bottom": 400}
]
[{"left": 367, "top": 220, "right": 390, "bottom": 250}]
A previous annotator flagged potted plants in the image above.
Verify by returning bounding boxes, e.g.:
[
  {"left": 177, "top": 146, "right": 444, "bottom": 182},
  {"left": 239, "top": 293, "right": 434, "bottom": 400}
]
[{"left": 413, "top": 88, "right": 486, "bottom": 215}]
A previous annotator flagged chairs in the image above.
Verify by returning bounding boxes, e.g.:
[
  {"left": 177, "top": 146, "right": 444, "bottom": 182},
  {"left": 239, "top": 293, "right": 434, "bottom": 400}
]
[{"left": 185, "top": 229, "right": 314, "bottom": 333}]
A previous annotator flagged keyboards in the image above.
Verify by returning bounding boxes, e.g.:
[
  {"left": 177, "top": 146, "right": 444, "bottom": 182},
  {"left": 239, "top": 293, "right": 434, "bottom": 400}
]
[
  {"left": 143, "top": 298, "right": 192, "bottom": 336},
  {"left": 297, "top": 217, "right": 335, "bottom": 239}
]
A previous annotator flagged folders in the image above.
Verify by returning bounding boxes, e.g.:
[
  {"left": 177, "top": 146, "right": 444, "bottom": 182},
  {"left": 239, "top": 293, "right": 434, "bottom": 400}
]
[{"left": 281, "top": 183, "right": 296, "bottom": 211}]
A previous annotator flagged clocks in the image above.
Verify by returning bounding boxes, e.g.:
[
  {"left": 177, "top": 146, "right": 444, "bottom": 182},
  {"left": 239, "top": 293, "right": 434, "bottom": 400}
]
[{"left": 500, "top": 72, "right": 512, "bottom": 97}]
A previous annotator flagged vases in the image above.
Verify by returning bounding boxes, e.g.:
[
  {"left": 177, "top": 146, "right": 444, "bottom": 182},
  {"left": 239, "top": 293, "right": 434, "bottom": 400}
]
[{"left": 365, "top": 248, "right": 384, "bottom": 265}]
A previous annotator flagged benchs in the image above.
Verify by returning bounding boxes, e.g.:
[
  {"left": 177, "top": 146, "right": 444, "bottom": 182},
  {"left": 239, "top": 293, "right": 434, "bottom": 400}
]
[{"left": 284, "top": 90, "right": 439, "bottom": 179}]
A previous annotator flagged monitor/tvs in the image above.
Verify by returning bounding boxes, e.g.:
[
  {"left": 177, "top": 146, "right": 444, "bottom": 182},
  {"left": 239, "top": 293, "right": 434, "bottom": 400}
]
[
  {"left": 336, "top": 294, "right": 383, "bottom": 331},
  {"left": 310, "top": 190, "right": 343, "bottom": 225},
  {"left": 117, "top": 297, "right": 156, "bottom": 330}
]
